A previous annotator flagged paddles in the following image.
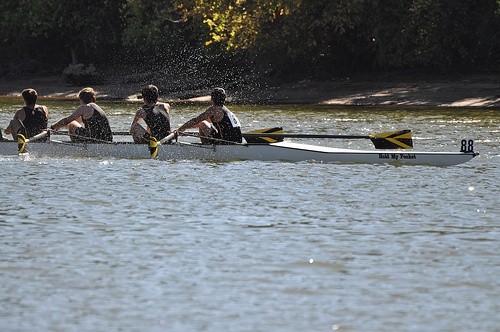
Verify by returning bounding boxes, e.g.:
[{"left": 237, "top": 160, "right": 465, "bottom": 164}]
[
  {"left": 18, "top": 130, "right": 47, "bottom": 153},
  {"left": 240, "top": 128, "right": 414, "bottom": 150},
  {"left": 149, "top": 131, "right": 176, "bottom": 158},
  {"left": 171, "top": 127, "right": 284, "bottom": 144}
]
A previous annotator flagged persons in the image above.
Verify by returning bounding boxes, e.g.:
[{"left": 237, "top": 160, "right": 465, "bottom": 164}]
[
  {"left": 174, "top": 87, "right": 242, "bottom": 145},
  {"left": 129, "top": 85, "right": 170, "bottom": 144},
  {"left": 4, "top": 89, "right": 48, "bottom": 142},
  {"left": 51, "top": 88, "right": 112, "bottom": 143}
]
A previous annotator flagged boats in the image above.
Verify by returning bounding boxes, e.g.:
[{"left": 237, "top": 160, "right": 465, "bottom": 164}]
[{"left": 0, "top": 139, "right": 482, "bottom": 167}]
